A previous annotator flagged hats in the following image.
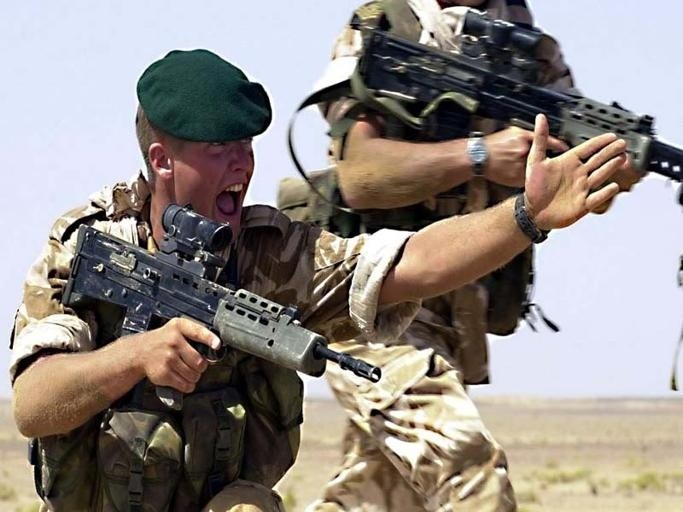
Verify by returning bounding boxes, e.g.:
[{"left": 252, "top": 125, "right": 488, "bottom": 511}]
[{"left": 137, "top": 50, "right": 272, "bottom": 142}]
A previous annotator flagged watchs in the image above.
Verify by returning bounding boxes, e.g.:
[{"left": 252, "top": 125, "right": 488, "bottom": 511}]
[{"left": 466, "top": 128, "right": 492, "bottom": 178}]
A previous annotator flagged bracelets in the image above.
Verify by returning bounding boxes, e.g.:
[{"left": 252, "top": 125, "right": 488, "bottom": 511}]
[{"left": 512, "top": 191, "right": 551, "bottom": 245}]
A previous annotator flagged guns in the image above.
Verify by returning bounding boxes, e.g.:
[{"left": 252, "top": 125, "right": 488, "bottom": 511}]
[
  {"left": 60, "top": 203, "right": 380, "bottom": 409},
  {"left": 358, "top": 11, "right": 682, "bottom": 205}
]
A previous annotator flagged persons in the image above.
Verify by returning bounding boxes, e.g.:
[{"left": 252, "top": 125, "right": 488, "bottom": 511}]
[
  {"left": 306, "top": 0, "right": 650, "bottom": 512},
  {"left": 8, "top": 46, "right": 632, "bottom": 511}
]
